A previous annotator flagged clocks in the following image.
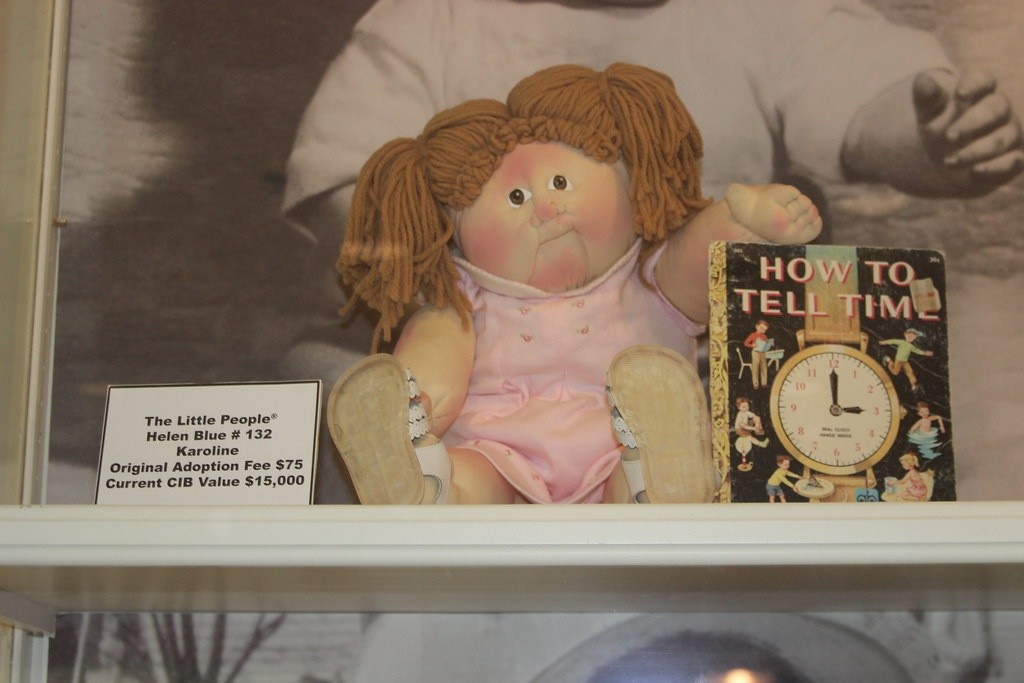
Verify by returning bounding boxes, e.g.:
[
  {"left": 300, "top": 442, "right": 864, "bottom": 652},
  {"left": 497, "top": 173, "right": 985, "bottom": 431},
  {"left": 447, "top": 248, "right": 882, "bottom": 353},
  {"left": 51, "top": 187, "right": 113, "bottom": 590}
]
[{"left": 766, "top": 342, "right": 909, "bottom": 480}]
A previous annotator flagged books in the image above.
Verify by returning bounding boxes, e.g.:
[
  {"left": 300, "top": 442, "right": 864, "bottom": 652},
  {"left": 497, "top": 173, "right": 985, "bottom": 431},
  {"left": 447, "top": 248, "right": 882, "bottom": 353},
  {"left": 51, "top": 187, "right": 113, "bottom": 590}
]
[{"left": 709, "top": 238, "right": 956, "bottom": 503}]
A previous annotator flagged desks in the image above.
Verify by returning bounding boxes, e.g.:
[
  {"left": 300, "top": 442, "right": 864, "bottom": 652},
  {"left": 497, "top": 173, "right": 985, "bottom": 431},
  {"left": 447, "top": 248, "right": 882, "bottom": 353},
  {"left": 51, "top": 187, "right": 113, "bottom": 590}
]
[{"left": 0, "top": 500, "right": 1024, "bottom": 613}]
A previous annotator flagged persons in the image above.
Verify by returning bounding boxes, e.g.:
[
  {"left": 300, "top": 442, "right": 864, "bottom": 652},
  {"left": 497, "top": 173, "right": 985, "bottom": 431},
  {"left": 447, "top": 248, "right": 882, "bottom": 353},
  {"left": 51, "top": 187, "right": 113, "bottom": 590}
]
[
  {"left": 327, "top": 59, "right": 822, "bottom": 502},
  {"left": 279, "top": 0, "right": 1024, "bottom": 349}
]
